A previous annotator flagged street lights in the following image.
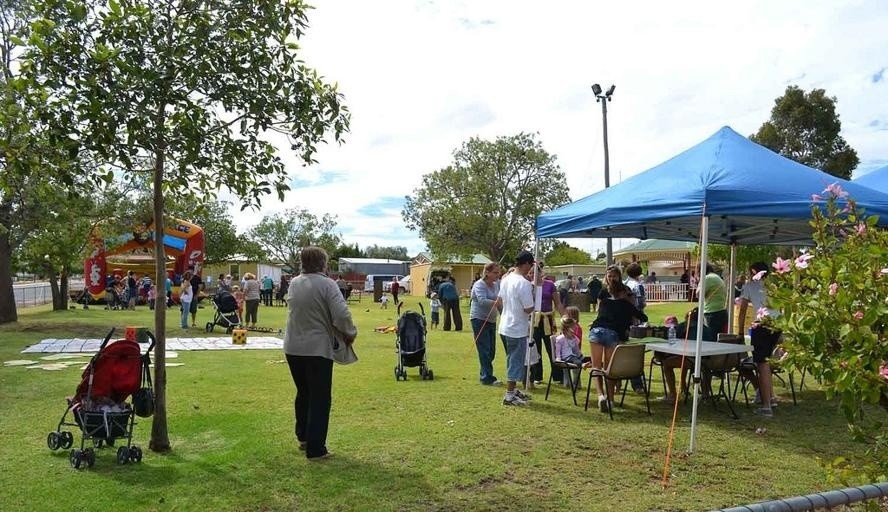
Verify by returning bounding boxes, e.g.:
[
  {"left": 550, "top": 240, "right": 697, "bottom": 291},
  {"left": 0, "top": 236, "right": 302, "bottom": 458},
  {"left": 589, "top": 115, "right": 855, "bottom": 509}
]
[{"left": 588, "top": 82, "right": 617, "bottom": 271}]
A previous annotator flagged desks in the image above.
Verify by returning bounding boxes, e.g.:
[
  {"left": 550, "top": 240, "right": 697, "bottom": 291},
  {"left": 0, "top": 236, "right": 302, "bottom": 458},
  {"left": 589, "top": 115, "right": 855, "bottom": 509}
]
[{"left": 566, "top": 291, "right": 591, "bottom": 313}]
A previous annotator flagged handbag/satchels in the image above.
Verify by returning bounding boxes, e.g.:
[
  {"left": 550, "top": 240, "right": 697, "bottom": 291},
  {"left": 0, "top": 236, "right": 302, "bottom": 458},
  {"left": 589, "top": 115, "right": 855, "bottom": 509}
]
[{"left": 132, "top": 388, "right": 154, "bottom": 416}]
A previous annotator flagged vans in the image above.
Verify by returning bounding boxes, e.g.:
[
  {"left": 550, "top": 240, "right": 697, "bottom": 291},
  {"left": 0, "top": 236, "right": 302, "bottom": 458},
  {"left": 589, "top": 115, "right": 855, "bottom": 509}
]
[{"left": 363, "top": 273, "right": 405, "bottom": 293}]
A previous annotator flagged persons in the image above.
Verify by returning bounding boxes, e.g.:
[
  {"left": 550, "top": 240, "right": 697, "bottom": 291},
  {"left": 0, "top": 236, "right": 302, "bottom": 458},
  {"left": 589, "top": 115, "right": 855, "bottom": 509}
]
[
  {"left": 283, "top": 246, "right": 359, "bottom": 461},
  {"left": 377, "top": 293, "right": 390, "bottom": 310},
  {"left": 103, "top": 265, "right": 350, "bottom": 331},
  {"left": 437, "top": 280, "right": 463, "bottom": 331},
  {"left": 470, "top": 251, "right": 787, "bottom": 415},
  {"left": 391, "top": 277, "right": 399, "bottom": 305},
  {"left": 430, "top": 291, "right": 442, "bottom": 330}
]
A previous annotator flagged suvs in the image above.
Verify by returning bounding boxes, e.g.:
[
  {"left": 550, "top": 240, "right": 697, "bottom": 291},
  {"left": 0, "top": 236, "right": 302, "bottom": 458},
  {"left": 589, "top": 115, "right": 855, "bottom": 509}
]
[{"left": 383, "top": 274, "right": 410, "bottom": 295}]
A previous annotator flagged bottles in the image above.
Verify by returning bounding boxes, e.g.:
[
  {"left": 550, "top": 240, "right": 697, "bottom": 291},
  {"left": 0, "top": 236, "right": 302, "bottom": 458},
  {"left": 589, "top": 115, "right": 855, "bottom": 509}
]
[{"left": 667, "top": 325, "right": 676, "bottom": 346}]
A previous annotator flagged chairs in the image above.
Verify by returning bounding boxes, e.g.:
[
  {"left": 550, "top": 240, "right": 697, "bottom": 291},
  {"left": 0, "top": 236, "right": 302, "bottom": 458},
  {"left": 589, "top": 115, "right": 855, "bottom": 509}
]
[{"left": 541, "top": 332, "right": 797, "bottom": 421}]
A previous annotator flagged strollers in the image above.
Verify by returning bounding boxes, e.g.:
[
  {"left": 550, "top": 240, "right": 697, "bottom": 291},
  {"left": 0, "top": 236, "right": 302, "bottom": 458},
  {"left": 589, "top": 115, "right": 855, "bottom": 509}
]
[
  {"left": 204, "top": 289, "right": 239, "bottom": 335},
  {"left": 107, "top": 287, "right": 128, "bottom": 311},
  {"left": 44, "top": 323, "right": 159, "bottom": 469},
  {"left": 393, "top": 300, "right": 434, "bottom": 383}
]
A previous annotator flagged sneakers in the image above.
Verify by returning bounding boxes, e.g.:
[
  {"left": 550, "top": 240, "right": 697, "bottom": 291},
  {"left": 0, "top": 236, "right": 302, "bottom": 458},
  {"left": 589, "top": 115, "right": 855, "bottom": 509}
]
[
  {"left": 753, "top": 407, "right": 773, "bottom": 417},
  {"left": 298, "top": 441, "right": 334, "bottom": 460},
  {"left": 656, "top": 394, "right": 675, "bottom": 404},
  {"left": 598, "top": 395, "right": 614, "bottom": 412},
  {"left": 770, "top": 397, "right": 777, "bottom": 407},
  {"left": 492, "top": 381, "right": 531, "bottom": 406}
]
